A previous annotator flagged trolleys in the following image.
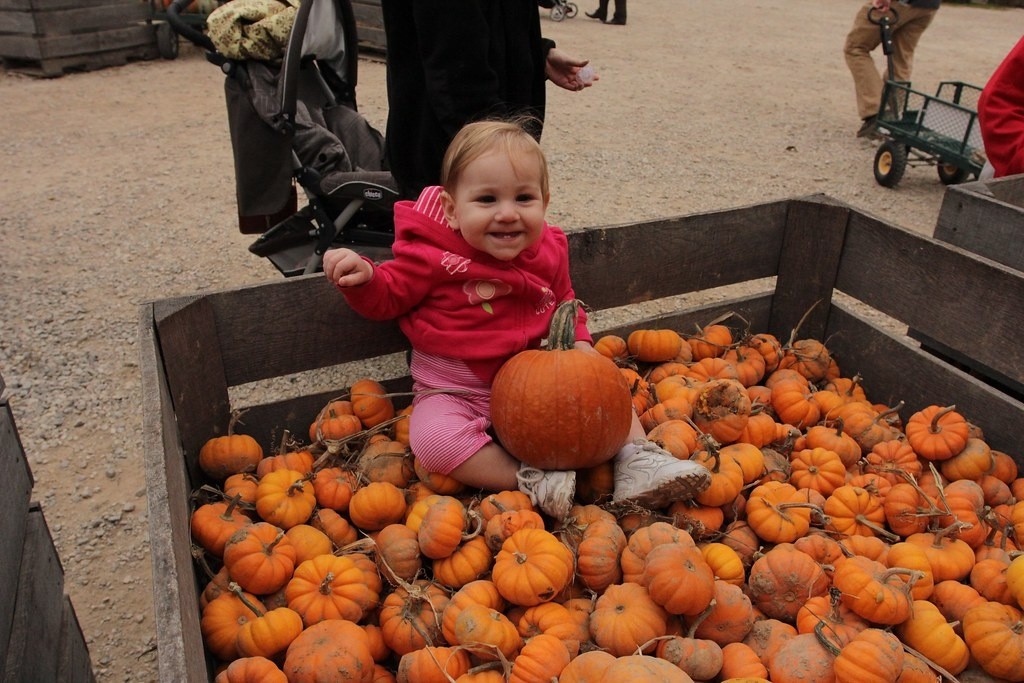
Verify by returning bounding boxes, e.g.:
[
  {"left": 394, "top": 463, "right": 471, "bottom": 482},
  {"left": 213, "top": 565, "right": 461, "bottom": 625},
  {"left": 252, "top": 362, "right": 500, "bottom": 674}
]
[
  {"left": 865, "top": 5, "right": 987, "bottom": 188},
  {"left": 136, "top": 0, "right": 1024, "bottom": 683}
]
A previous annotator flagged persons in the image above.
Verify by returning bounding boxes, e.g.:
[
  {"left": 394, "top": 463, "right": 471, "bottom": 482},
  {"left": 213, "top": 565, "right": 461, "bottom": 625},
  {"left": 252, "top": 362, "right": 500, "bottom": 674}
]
[
  {"left": 323, "top": 119, "right": 712, "bottom": 522},
  {"left": 381, "top": 1, "right": 600, "bottom": 200},
  {"left": 845, "top": 0, "right": 940, "bottom": 138},
  {"left": 584, "top": 0, "right": 626, "bottom": 25},
  {"left": 977, "top": 33, "right": 1024, "bottom": 177}
]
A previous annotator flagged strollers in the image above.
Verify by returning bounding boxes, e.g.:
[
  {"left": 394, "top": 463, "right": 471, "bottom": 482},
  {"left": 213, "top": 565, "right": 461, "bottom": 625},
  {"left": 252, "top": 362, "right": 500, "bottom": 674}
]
[
  {"left": 536, "top": 0, "right": 579, "bottom": 21},
  {"left": 166, "top": 0, "right": 406, "bottom": 277}
]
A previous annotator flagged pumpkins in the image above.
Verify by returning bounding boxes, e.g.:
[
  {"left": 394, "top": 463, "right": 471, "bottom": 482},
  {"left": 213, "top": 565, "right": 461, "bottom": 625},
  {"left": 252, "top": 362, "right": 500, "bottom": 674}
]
[{"left": 190, "top": 299, "right": 1024, "bottom": 683}]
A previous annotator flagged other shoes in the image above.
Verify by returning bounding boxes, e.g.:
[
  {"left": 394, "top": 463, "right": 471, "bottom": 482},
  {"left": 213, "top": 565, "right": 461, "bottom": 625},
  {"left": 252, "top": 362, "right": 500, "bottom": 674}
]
[
  {"left": 606, "top": 19, "right": 625, "bottom": 24},
  {"left": 857, "top": 111, "right": 892, "bottom": 136},
  {"left": 585, "top": 11, "right": 607, "bottom": 21}
]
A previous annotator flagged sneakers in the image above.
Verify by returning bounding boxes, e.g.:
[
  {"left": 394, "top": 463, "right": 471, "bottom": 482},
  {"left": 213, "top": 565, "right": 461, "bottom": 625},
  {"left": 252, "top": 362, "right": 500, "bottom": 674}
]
[
  {"left": 612, "top": 437, "right": 711, "bottom": 510},
  {"left": 515, "top": 462, "right": 576, "bottom": 521}
]
